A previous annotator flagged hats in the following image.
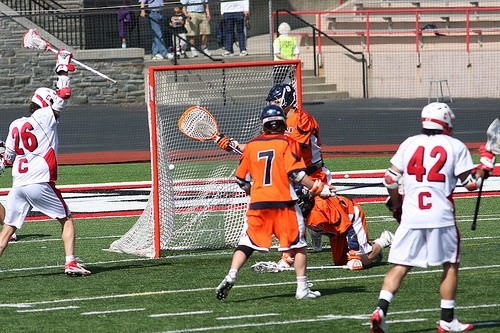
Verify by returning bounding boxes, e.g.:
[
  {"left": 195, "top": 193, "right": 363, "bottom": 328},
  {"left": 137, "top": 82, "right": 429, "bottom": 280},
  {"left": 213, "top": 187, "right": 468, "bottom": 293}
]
[{"left": 278, "top": 22, "right": 290, "bottom": 35}]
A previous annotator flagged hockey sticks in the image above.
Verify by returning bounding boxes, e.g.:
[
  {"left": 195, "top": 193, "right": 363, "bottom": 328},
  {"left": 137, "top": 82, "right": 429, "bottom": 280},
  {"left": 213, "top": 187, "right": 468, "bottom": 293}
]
[
  {"left": 177, "top": 106, "right": 242, "bottom": 155},
  {"left": 471, "top": 118, "right": 500, "bottom": 230},
  {"left": 22, "top": 27, "right": 118, "bottom": 85},
  {"left": 332, "top": 178, "right": 406, "bottom": 196},
  {"left": 251, "top": 262, "right": 349, "bottom": 274}
]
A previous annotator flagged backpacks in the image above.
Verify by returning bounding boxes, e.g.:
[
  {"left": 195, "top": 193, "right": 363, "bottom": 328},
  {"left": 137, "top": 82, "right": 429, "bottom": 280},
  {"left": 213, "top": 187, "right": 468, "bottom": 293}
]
[{"left": 216, "top": 18, "right": 225, "bottom": 48}]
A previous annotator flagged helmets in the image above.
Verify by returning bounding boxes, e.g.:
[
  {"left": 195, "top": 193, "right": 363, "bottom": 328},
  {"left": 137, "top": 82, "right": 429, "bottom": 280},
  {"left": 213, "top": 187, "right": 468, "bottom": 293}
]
[
  {"left": 260, "top": 104, "right": 286, "bottom": 130},
  {"left": 31, "top": 87, "right": 56, "bottom": 109},
  {"left": 266, "top": 83, "right": 297, "bottom": 110},
  {"left": 421, "top": 102, "right": 455, "bottom": 136}
]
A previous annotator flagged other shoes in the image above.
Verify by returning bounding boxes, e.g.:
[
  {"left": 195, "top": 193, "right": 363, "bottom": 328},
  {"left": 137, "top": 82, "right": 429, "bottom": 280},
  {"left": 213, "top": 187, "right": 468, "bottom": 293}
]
[{"left": 176, "top": 51, "right": 187, "bottom": 59}]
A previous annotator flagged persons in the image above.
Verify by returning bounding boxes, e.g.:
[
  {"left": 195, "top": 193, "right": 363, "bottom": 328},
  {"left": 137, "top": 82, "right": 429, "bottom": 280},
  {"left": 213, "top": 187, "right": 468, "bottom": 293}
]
[
  {"left": 0, "top": 48, "right": 91, "bottom": 276},
  {"left": 180, "top": 0, "right": 212, "bottom": 56},
  {"left": 257, "top": 186, "right": 395, "bottom": 271},
  {"left": 273, "top": 23, "right": 300, "bottom": 86},
  {"left": 369, "top": 102, "right": 495, "bottom": 333},
  {"left": 215, "top": 105, "right": 336, "bottom": 300},
  {"left": 139, "top": 0, "right": 175, "bottom": 60},
  {"left": 220, "top": 0, "right": 249, "bottom": 56},
  {"left": 214, "top": 83, "right": 332, "bottom": 251},
  {"left": 168, "top": 5, "right": 188, "bottom": 59},
  {"left": 115, "top": 0, "right": 137, "bottom": 48}
]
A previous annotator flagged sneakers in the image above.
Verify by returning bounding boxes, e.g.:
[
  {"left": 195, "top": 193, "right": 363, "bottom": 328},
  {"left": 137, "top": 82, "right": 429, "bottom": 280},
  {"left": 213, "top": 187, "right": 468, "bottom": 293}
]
[
  {"left": 9, "top": 233, "right": 17, "bottom": 241},
  {"left": 152, "top": 54, "right": 164, "bottom": 60},
  {"left": 438, "top": 318, "right": 473, "bottom": 333},
  {"left": 370, "top": 307, "right": 385, "bottom": 333},
  {"left": 296, "top": 287, "right": 321, "bottom": 300},
  {"left": 381, "top": 230, "right": 395, "bottom": 247},
  {"left": 204, "top": 50, "right": 212, "bottom": 56},
  {"left": 240, "top": 51, "right": 248, "bottom": 56},
  {"left": 222, "top": 52, "right": 234, "bottom": 56},
  {"left": 215, "top": 274, "right": 237, "bottom": 300},
  {"left": 191, "top": 50, "right": 198, "bottom": 57},
  {"left": 65, "top": 259, "right": 91, "bottom": 277},
  {"left": 373, "top": 236, "right": 383, "bottom": 263},
  {"left": 165, "top": 53, "right": 173, "bottom": 59}
]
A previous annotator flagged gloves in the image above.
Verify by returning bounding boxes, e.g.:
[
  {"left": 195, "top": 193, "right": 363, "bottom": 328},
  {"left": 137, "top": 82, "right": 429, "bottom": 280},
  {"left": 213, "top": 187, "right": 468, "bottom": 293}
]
[
  {"left": 309, "top": 179, "right": 336, "bottom": 199},
  {"left": 216, "top": 134, "right": 239, "bottom": 153},
  {"left": 384, "top": 193, "right": 403, "bottom": 223},
  {"left": 54, "top": 48, "right": 73, "bottom": 74},
  {"left": 478, "top": 143, "right": 496, "bottom": 178}
]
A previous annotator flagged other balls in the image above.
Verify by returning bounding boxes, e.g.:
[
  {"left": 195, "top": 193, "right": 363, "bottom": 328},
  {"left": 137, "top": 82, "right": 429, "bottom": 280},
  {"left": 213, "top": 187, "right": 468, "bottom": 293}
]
[{"left": 169, "top": 164, "right": 175, "bottom": 170}]
